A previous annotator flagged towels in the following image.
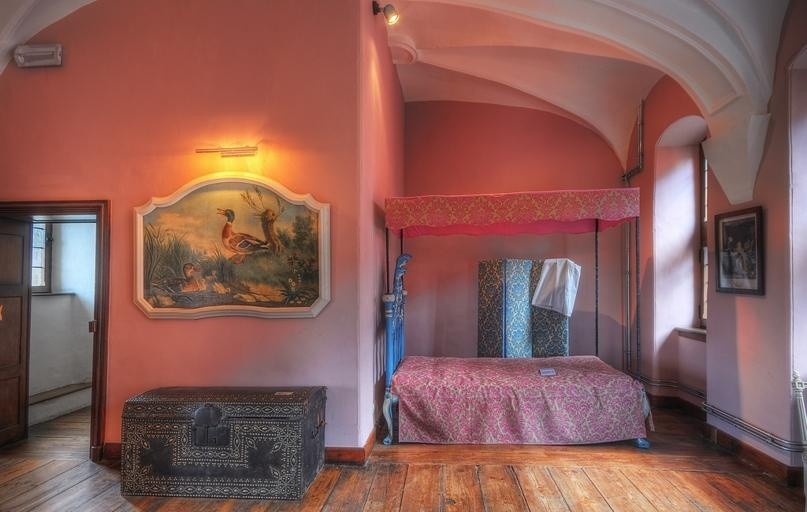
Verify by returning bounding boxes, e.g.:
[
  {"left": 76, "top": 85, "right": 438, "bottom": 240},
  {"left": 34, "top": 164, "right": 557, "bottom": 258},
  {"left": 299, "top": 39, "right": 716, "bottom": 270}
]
[{"left": 530, "top": 258, "right": 583, "bottom": 317}]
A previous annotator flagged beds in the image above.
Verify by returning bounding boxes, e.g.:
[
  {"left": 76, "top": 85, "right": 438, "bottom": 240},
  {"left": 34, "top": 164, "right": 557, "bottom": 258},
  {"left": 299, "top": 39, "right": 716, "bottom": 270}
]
[{"left": 379, "top": 250, "right": 656, "bottom": 452}]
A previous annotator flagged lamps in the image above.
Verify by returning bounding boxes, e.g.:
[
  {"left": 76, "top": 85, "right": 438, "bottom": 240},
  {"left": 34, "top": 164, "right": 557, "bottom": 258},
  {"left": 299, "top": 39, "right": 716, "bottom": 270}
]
[
  {"left": 193, "top": 143, "right": 261, "bottom": 161},
  {"left": 11, "top": 41, "right": 65, "bottom": 69},
  {"left": 371, "top": 0, "right": 401, "bottom": 27}
]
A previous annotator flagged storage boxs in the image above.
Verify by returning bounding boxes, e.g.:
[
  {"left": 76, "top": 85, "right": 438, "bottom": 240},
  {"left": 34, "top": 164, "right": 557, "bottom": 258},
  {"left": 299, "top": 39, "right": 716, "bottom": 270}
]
[{"left": 118, "top": 384, "right": 329, "bottom": 503}]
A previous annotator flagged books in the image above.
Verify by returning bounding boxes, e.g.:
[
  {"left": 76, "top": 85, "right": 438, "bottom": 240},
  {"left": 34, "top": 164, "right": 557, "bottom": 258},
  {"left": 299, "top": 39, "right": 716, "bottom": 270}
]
[{"left": 538, "top": 367, "right": 554, "bottom": 377}]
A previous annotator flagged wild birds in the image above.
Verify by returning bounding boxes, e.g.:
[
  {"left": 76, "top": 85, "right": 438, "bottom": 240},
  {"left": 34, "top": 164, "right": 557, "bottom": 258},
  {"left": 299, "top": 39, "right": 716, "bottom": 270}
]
[
  {"left": 216, "top": 208, "right": 270, "bottom": 266},
  {"left": 150, "top": 262, "right": 201, "bottom": 294}
]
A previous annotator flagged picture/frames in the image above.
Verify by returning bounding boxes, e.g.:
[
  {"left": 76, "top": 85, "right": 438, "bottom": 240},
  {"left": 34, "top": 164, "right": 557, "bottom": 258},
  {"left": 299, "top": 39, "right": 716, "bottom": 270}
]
[
  {"left": 712, "top": 204, "right": 766, "bottom": 297},
  {"left": 130, "top": 169, "right": 333, "bottom": 324}
]
